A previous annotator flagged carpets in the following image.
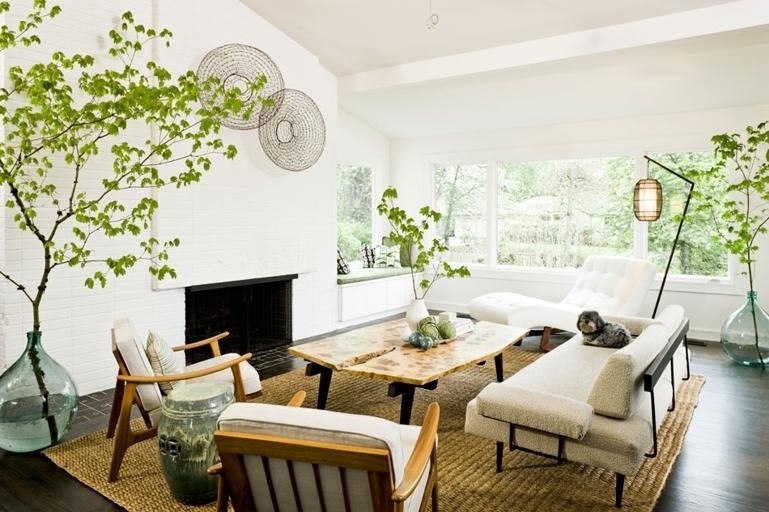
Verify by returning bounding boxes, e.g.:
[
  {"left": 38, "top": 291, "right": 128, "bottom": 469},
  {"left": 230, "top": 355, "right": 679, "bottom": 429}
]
[{"left": 42, "top": 345, "right": 706, "bottom": 512}]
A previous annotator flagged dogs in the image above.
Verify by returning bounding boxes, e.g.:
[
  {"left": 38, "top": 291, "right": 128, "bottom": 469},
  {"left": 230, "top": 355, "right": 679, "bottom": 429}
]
[{"left": 576, "top": 311, "right": 632, "bottom": 349}]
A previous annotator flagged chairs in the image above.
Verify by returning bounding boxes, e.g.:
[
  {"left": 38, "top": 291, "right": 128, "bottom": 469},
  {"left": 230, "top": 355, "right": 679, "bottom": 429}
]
[
  {"left": 471, "top": 254, "right": 658, "bottom": 351},
  {"left": 207, "top": 390, "right": 440, "bottom": 512},
  {"left": 106, "top": 316, "right": 263, "bottom": 482}
]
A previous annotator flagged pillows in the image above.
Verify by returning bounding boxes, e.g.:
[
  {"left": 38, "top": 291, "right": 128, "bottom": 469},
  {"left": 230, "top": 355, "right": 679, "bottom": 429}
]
[
  {"left": 336, "top": 246, "right": 351, "bottom": 274},
  {"left": 361, "top": 236, "right": 413, "bottom": 268},
  {"left": 146, "top": 329, "right": 186, "bottom": 396}
]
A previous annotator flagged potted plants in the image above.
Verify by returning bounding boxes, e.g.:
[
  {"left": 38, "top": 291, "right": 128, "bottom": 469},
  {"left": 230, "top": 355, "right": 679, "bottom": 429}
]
[
  {"left": 0, "top": 0, "right": 277, "bottom": 454},
  {"left": 670, "top": 120, "right": 769, "bottom": 369},
  {"left": 376, "top": 185, "right": 472, "bottom": 333}
]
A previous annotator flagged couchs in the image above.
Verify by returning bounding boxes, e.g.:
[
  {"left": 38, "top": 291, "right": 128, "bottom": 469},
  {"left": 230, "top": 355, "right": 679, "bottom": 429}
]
[{"left": 464, "top": 304, "right": 691, "bottom": 508}]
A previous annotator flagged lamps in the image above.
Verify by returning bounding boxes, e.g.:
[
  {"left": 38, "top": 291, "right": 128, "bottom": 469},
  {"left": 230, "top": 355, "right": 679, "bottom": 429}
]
[{"left": 634, "top": 156, "right": 695, "bottom": 319}]
